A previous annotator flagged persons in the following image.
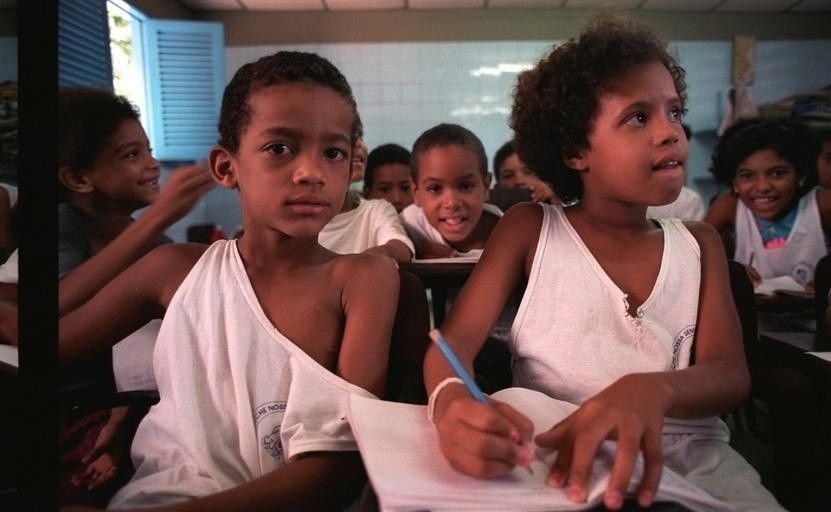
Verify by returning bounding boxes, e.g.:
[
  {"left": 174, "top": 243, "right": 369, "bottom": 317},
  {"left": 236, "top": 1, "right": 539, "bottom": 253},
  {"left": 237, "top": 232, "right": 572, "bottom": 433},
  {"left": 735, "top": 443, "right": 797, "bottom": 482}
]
[
  {"left": 54, "top": 51, "right": 401, "bottom": 510},
  {"left": 424, "top": 9, "right": 783, "bottom": 512},
  {"left": 317, "top": 113, "right": 830, "bottom": 450},
  {"left": 1, "top": 82, "right": 221, "bottom": 511}
]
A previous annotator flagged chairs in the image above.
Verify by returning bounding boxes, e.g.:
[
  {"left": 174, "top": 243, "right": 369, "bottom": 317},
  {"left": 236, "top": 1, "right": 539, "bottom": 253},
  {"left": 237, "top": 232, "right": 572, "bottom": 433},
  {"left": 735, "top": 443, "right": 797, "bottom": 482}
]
[{"left": 58, "top": 269, "right": 429, "bottom": 512}]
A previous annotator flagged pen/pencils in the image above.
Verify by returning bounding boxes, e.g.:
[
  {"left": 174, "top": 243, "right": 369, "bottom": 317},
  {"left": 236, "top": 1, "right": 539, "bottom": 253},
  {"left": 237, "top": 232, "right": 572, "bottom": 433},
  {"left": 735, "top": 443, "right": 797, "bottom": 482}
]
[
  {"left": 747, "top": 250, "right": 756, "bottom": 266},
  {"left": 425, "top": 324, "right": 538, "bottom": 477}
]
